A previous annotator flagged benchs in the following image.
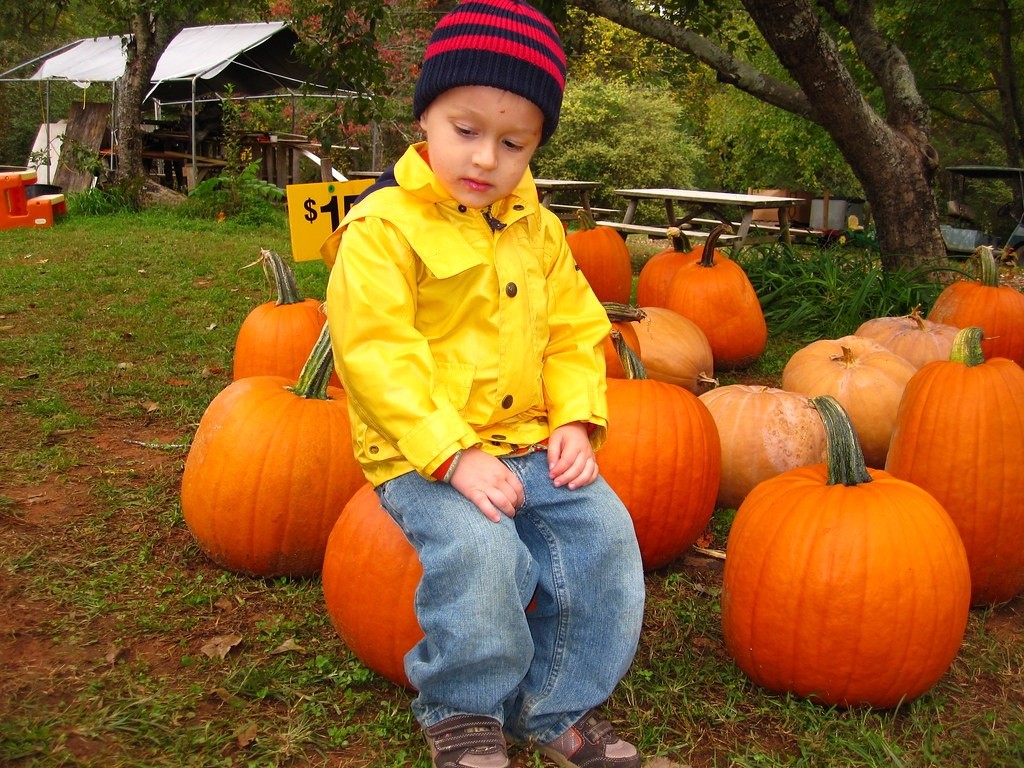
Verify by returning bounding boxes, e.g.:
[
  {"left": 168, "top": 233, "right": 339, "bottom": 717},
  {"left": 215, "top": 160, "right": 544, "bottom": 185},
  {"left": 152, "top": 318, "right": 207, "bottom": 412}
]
[
  {"left": 540, "top": 203, "right": 621, "bottom": 217},
  {"left": 673, "top": 216, "right": 824, "bottom": 240},
  {"left": 592, "top": 219, "right": 744, "bottom": 246}
]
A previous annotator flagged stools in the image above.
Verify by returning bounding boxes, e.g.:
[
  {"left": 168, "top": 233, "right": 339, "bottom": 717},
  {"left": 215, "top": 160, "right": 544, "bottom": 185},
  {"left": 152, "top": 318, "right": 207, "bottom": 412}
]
[{"left": 27, "top": 193, "right": 69, "bottom": 227}]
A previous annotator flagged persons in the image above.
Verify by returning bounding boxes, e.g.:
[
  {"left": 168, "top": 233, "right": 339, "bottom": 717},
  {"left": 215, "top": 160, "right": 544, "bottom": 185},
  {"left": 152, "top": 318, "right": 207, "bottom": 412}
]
[{"left": 319, "top": 0, "right": 645, "bottom": 768}]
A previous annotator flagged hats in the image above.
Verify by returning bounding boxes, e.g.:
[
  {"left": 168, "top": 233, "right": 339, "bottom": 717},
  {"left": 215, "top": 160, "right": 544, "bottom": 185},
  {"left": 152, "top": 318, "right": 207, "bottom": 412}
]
[{"left": 412, "top": 0, "right": 567, "bottom": 148}]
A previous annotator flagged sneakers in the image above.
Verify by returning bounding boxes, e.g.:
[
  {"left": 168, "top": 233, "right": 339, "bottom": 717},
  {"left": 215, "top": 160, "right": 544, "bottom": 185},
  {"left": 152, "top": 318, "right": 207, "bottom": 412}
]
[
  {"left": 502, "top": 708, "right": 642, "bottom": 768},
  {"left": 422, "top": 714, "right": 512, "bottom": 768}
]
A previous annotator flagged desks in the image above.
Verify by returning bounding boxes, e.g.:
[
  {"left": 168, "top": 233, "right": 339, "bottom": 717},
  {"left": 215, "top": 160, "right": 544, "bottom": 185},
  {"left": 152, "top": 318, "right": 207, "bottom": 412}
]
[
  {"left": 531, "top": 177, "right": 605, "bottom": 227},
  {"left": 613, "top": 188, "right": 805, "bottom": 261},
  {"left": 0, "top": 171, "right": 38, "bottom": 228}
]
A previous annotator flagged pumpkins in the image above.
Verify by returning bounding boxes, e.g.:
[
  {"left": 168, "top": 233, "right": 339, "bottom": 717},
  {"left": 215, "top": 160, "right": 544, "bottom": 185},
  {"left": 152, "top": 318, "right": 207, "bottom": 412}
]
[{"left": 181, "top": 209, "right": 1024, "bottom": 709}]
